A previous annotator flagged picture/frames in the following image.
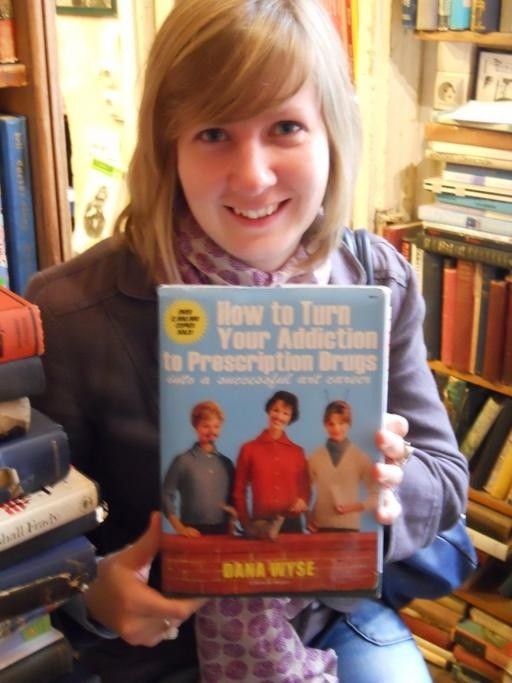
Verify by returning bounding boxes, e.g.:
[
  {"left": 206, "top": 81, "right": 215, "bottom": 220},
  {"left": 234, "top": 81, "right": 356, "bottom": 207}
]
[{"left": 153, "top": 0, "right": 363, "bottom": 103}]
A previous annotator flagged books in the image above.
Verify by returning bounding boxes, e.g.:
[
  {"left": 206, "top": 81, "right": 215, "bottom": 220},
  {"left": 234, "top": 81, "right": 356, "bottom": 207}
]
[
  {"left": 152, "top": 283, "right": 396, "bottom": 606},
  {"left": 370, "top": 2, "right": 511, "bottom": 683},
  {"left": 2, "top": 0, "right": 104, "bottom": 683}
]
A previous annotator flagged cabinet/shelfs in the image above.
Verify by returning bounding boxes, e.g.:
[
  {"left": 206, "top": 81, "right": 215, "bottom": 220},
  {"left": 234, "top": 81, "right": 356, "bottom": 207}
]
[
  {"left": 2, "top": 0, "right": 63, "bottom": 297},
  {"left": 379, "top": 0, "right": 512, "bottom": 682}
]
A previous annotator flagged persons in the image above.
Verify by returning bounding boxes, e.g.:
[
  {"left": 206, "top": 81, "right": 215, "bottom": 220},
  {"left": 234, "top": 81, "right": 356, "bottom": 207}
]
[
  {"left": 302, "top": 396, "right": 382, "bottom": 535},
  {"left": 161, "top": 398, "right": 238, "bottom": 539},
  {"left": 23, "top": 0, "right": 480, "bottom": 683},
  {"left": 230, "top": 386, "right": 313, "bottom": 539}
]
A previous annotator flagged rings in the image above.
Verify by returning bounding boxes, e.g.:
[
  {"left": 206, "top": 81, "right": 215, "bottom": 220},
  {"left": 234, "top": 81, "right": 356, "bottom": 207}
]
[{"left": 161, "top": 618, "right": 172, "bottom": 632}]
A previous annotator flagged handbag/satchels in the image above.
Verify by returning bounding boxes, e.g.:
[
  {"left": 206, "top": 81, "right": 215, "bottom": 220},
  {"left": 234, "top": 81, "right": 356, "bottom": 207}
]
[{"left": 244, "top": 515, "right": 284, "bottom": 543}]
